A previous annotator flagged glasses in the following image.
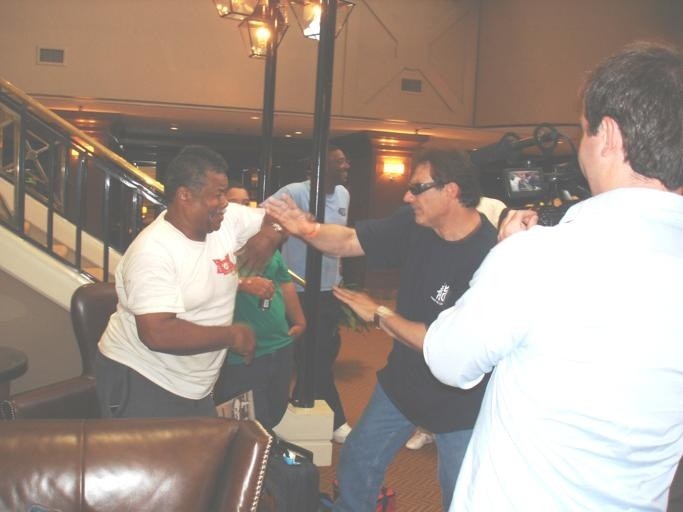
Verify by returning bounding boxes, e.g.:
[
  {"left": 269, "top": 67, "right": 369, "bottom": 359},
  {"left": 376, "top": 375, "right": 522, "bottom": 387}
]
[{"left": 406, "top": 181, "right": 445, "bottom": 194}]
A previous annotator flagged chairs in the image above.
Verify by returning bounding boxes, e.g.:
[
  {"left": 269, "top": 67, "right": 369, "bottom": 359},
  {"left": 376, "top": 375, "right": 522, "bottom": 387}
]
[{"left": 0, "top": 283, "right": 271, "bottom": 511}]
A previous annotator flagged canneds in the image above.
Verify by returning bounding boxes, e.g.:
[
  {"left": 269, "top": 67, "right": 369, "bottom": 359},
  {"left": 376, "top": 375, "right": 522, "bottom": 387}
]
[{"left": 259, "top": 298, "right": 270, "bottom": 310}]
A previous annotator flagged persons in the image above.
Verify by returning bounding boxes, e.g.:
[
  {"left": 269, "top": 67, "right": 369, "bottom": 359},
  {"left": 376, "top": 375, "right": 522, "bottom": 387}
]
[{"left": 97, "top": 41, "right": 683, "bottom": 511}]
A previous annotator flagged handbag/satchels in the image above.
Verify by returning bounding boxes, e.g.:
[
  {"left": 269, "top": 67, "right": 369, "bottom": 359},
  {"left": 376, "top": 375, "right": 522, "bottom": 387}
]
[{"left": 258, "top": 438, "right": 320, "bottom": 512}]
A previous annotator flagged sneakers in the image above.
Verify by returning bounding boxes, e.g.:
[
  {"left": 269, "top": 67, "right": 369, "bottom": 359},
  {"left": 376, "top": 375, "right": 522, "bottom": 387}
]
[
  {"left": 331, "top": 422, "right": 352, "bottom": 443},
  {"left": 405, "top": 430, "right": 434, "bottom": 450}
]
